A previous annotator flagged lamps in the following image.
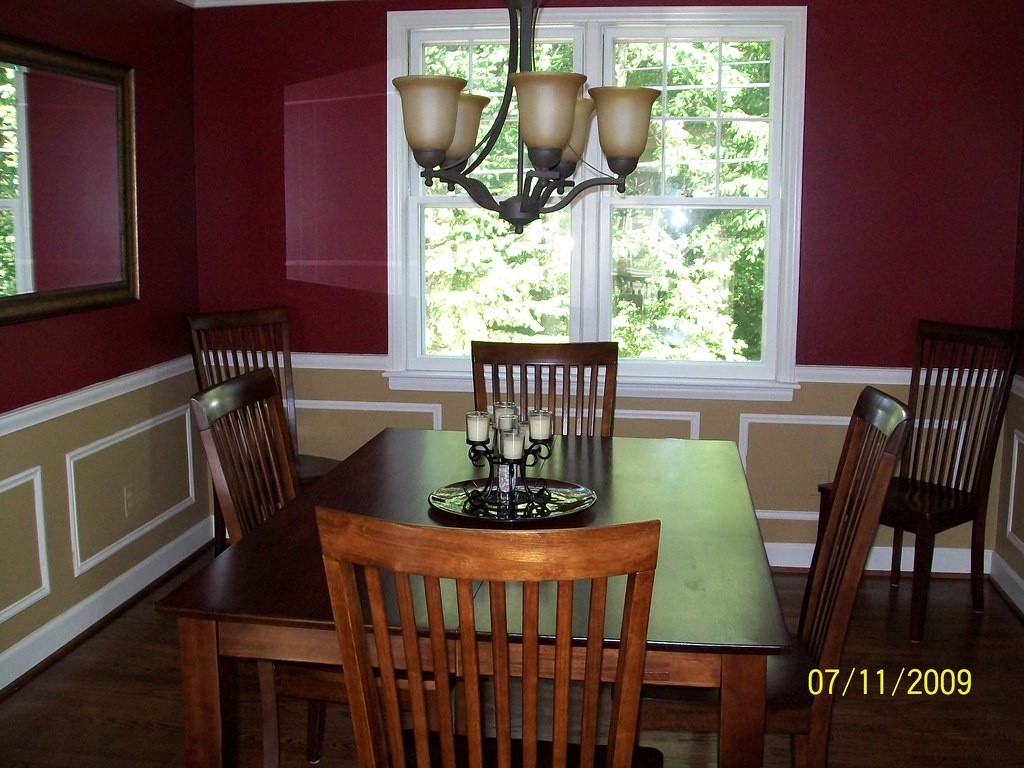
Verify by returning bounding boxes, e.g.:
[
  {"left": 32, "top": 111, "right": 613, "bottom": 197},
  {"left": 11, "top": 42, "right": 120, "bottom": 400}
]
[
  {"left": 459, "top": 402, "right": 553, "bottom": 520},
  {"left": 390, "top": 0, "right": 662, "bottom": 234}
]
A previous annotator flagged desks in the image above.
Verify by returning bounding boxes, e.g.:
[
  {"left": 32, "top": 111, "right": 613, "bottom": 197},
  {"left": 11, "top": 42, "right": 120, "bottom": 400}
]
[{"left": 151, "top": 426, "right": 795, "bottom": 768}]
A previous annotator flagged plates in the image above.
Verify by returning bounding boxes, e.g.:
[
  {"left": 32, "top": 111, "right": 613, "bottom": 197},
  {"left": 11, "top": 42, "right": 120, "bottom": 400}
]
[{"left": 428, "top": 477, "right": 598, "bottom": 524}]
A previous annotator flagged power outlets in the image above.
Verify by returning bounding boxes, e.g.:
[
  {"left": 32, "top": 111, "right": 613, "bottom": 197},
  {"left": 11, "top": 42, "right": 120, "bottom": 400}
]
[{"left": 811, "top": 467, "right": 830, "bottom": 495}]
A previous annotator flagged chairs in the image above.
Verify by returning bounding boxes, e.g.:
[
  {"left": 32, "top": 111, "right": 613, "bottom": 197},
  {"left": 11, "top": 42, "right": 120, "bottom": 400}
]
[
  {"left": 181, "top": 307, "right": 341, "bottom": 561},
  {"left": 817, "top": 317, "right": 1023, "bottom": 646},
  {"left": 313, "top": 504, "right": 666, "bottom": 768},
  {"left": 191, "top": 367, "right": 462, "bottom": 768},
  {"left": 612, "top": 386, "right": 911, "bottom": 768},
  {"left": 472, "top": 342, "right": 619, "bottom": 438}
]
